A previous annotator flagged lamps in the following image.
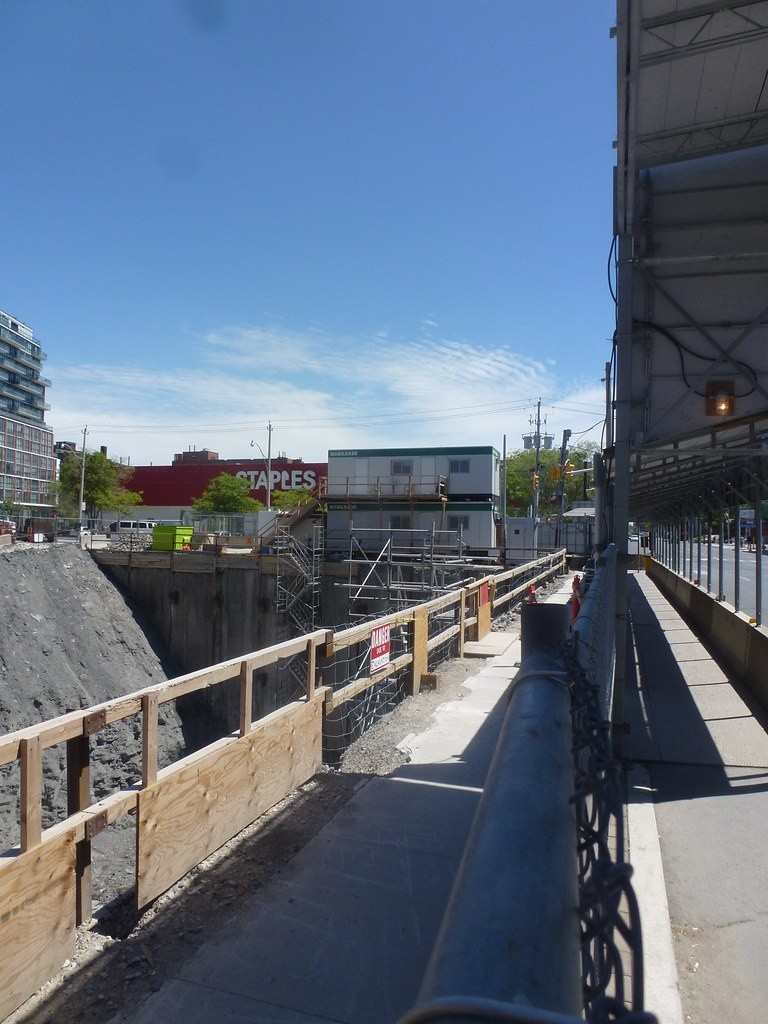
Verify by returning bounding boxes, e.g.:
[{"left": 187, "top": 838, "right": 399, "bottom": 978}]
[{"left": 704, "top": 389, "right": 734, "bottom": 418}]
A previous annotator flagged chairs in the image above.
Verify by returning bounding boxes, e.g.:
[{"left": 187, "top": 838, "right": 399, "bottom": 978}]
[
  {"left": 369, "top": 483, "right": 382, "bottom": 494},
  {"left": 403, "top": 483, "right": 416, "bottom": 494}
]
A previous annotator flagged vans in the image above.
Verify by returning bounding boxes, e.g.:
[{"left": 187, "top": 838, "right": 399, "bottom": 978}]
[{"left": 108, "top": 520, "right": 157, "bottom": 534}]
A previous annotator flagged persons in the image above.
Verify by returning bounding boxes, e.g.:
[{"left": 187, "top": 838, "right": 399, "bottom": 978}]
[
  {"left": 747, "top": 536, "right": 752, "bottom": 549},
  {"left": 740, "top": 535, "right": 745, "bottom": 548}
]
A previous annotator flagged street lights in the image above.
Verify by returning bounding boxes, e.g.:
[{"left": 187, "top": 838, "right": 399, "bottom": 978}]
[
  {"left": 250, "top": 420, "right": 273, "bottom": 512},
  {"left": 60, "top": 425, "right": 90, "bottom": 542}
]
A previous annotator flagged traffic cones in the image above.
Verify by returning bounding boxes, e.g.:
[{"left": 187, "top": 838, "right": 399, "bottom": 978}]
[{"left": 527, "top": 582, "right": 537, "bottom": 605}]
